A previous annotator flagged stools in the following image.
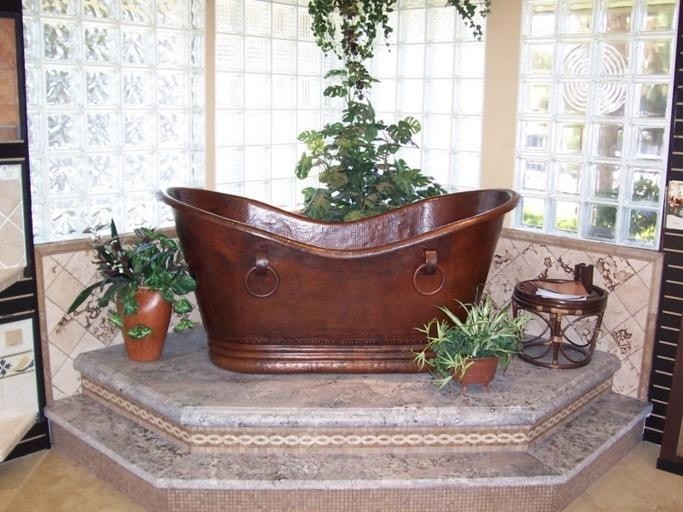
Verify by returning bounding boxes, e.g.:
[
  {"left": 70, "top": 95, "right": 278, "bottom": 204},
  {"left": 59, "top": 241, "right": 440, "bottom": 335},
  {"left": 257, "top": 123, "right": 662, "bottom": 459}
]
[{"left": 515, "top": 277, "right": 606, "bottom": 370}]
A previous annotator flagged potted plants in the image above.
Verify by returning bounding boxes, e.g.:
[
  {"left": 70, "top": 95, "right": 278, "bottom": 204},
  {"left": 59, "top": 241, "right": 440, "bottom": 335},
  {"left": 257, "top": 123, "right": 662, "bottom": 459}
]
[
  {"left": 411, "top": 297, "right": 528, "bottom": 387},
  {"left": 54, "top": 220, "right": 198, "bottom": 362}
]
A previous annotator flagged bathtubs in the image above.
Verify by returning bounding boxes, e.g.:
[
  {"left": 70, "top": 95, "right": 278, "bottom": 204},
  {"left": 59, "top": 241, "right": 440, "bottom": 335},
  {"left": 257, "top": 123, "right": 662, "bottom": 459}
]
[{"left": 156, "top": 188, "right": 520, "bottom": 374}]
